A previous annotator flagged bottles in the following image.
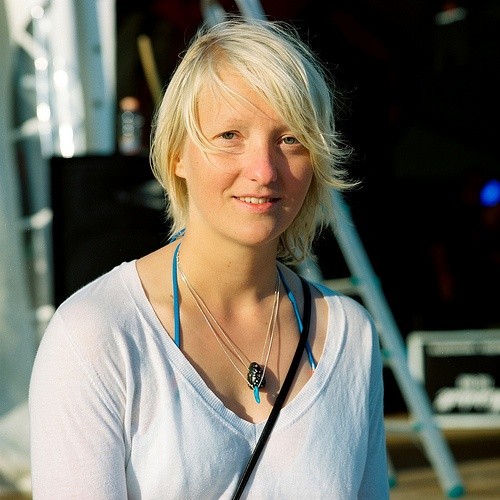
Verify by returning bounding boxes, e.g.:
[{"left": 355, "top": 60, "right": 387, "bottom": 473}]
[{"left": 117, "top": 96, "right": 145, "bottom": 155}]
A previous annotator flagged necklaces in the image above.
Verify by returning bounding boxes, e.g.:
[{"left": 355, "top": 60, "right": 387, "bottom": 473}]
[{"left": 172, "top": 236, "right": 283, "bottom": 406}]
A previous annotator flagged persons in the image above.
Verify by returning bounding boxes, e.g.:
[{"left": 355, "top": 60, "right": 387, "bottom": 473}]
[{"left": 24, "top": 17, "right": 393, "bottom": 500}]
